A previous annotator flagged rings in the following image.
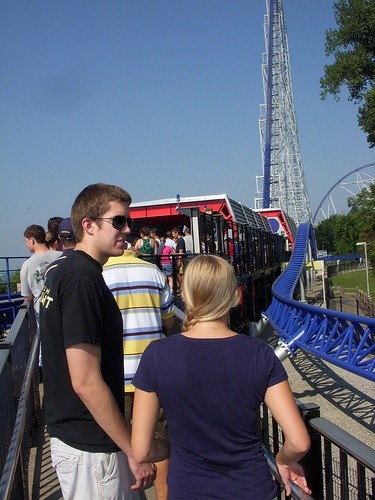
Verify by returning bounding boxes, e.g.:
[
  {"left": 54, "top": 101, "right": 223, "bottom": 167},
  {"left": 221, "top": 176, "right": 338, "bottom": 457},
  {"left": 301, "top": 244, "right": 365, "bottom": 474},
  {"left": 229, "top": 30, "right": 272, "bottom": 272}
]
[{"left": 283, "top": 480, "right": 290, "bottom": 487}]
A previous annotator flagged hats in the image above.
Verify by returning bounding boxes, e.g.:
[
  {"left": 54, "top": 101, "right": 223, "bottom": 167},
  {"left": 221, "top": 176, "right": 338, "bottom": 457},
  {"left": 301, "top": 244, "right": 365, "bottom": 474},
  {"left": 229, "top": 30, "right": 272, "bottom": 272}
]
[{"left": 58, "top": 218, "right": 75, "bottom": 241}]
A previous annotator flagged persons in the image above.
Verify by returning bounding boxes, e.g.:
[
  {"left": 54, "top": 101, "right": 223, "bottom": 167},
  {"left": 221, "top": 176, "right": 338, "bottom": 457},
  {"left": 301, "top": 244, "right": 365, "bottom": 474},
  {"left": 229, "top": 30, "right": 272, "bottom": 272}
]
[
  {"left": 131, "top": 255, "right": 312, "bottom": 500},
  {"left": 40, "top": 182, "right": 158, "bottom": 500},
  {"left": 19, "top": 218, "right": 195, "bottom": 393}
]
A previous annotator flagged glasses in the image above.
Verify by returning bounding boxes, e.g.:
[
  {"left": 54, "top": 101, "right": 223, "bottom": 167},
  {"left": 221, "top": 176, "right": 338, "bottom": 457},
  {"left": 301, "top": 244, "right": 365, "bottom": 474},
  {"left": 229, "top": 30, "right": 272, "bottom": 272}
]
[{"left": 91, "top": 216, "right": 133, "bottom": 230}]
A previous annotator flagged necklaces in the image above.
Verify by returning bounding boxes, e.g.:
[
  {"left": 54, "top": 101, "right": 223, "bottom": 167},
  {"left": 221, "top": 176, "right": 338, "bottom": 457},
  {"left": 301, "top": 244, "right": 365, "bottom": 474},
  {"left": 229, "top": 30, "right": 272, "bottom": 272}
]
[{"left": 193, "top": 324, "right": 229, "bottom": 331}]
